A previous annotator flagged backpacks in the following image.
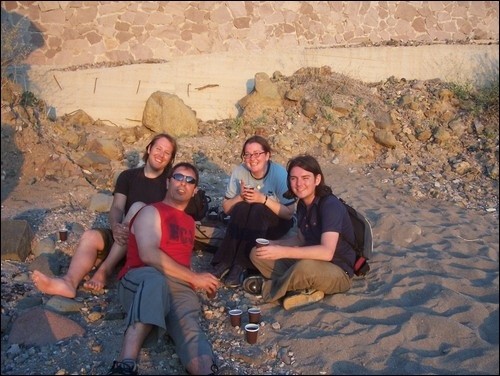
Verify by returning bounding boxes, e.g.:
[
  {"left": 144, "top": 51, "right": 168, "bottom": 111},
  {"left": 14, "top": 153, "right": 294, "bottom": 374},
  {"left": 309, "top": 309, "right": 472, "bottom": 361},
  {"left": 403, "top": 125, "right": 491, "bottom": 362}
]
[
  {"left": 184, "top": 190, "right": 211, "bottom": 222},
  {"left": 298, "top": 193, "right": 373, "bottom": 276}
]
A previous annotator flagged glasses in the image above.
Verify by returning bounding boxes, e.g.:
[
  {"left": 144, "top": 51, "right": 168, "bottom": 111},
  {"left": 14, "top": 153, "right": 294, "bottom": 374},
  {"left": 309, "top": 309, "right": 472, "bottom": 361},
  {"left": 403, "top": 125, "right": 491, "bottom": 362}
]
[
  {"left": 243, "top": 152, "right": 265, "bottom": 159},
  {"left": 172, "top": 174, "right": 197, "bottom": 184}
]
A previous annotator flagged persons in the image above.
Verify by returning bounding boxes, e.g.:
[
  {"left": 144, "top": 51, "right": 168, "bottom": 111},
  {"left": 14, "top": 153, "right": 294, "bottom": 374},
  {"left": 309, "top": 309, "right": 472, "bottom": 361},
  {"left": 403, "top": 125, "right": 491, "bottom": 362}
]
[
  {"left": 210, "top": 136, "right": 297, "bottom": 287},
  {"left": 249, "top": 154, "right": 356, "bottom": 312},
  {"left": 32, "top": 134, "right": 178, "bottom": 298},
  {"left": 106, "top": 162, "right": 221, "bottom": 376}
]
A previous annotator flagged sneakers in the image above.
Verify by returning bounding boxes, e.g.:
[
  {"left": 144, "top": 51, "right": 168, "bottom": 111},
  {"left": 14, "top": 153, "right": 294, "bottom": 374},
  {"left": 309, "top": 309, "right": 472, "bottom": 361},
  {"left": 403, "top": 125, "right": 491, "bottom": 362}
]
[
  {"left": 207, "top": 261, "right": 230, "bottom": 279},
  {"left": 225, "top": 263, "right": 248, "bottom": 287},
  {"left": 208, "top": 365, "right": 222, "bottom": 376},
  {"left": 219, "top": 212, "right": 231, "bottom": 225},
  {"left": 283, "top": 288, "right": 324, "bottom": 311},
  {"left": 105, "top": 359, "right": 138, "bottom": 376},
  {"left": 243, "top": 276, "right": 264, "bottom": 296},
  {"left": 208, "top": 207, "right": 218, "bottom": 221}
]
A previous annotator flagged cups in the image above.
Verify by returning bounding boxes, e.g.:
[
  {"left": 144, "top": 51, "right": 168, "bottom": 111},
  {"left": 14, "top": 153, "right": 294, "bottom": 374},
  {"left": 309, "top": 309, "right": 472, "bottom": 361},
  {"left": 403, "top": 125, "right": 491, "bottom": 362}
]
[
  {"left": 228, "top": 309, "right": 243, "bottom": 327},
  {"left": 59, "top": 230, "right": 67, "bottom": 241},
  {"left": 244, "top": 324, "right": 260, "bottom": 346},
  {"left": 255, "top": 238, "right": 269, "bottom": 249},
  {"left": 243, "top": 185, "right": 254, "bottom": 202},
  {"left": 248, "top": 308, "right": 261, "bottom": 323},
  {"left": 207, "top": 283, "right": 218, "bottom": 299}
]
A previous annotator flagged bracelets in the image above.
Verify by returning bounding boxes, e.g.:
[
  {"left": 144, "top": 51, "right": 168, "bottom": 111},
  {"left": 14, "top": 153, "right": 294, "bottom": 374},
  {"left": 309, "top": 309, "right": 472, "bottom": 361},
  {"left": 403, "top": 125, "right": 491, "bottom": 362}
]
[{"left": 263, "top": 195, "right": 268, "bottom": 205}]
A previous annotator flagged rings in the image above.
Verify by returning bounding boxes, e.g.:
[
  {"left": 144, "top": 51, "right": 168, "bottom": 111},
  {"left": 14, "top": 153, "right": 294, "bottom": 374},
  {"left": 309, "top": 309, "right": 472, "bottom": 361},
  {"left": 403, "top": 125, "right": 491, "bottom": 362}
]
[{"left": 123, "top": 226, "right": 126, "bottom": 230}]
